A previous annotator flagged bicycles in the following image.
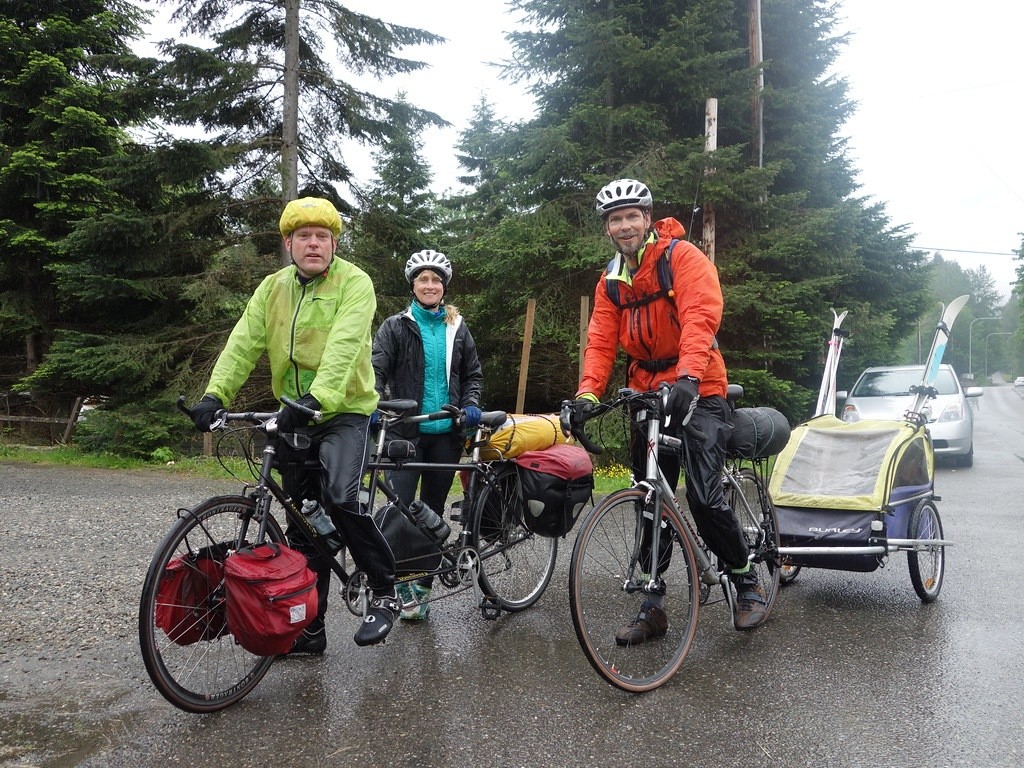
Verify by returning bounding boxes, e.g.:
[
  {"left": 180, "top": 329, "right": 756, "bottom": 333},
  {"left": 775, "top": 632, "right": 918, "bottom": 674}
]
[
  {"left": 139, "top": 395, "right": 559, "bottom": 714},
  {"left": 560, "top": 381, "right": 782, "bottom": 693}
]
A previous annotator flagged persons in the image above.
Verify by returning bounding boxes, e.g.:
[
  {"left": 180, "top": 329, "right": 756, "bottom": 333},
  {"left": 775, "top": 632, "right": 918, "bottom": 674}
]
[
  {"left": 370, "top": 250, "right": 484, "bottom": 621},
  {"left": 190, "top": 196, "right": 403, "bottom": 657},
  {"left": 559, "top": 178, "right": 768, "bottom": 646}
]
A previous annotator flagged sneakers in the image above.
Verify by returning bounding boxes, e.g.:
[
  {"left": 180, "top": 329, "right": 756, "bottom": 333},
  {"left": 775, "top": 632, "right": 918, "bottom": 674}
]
[
  {"left": 285, "top": 627, "right": 326, "bottom": 655},
  {"left": 617, "top": 601, "right": 668, "bottom": 645},
  {"left": 392, "top": 582, "right": 414, "bottom": 606},
  {"left": 354, "top": 589, "right": 401, "bottom": 646},
  {"left": 734, "top": 582, "right": 767, "bottom": 631},
  {"left": 401, "top": 585, "right": 433, "bottom": 619}
]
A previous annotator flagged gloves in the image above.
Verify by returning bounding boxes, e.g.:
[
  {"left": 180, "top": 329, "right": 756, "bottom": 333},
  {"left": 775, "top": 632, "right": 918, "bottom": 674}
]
[
  {"left": 190, "top": 394, "right": 224, "bottom": 432},
  {"left": 276, "top": 393, "right": 322, "bottom": 434},
  {"left": 371, "top": 412, "right": 379, "bottom": 423},
  {"left": 569, "top": 398, "right": 595, "bottom": 435},
  {"left": 460, "top": 406, "right": 482, "bottom": 427},
  {"left": 662, "top": 376, "right": 700, "bottom": 425}
]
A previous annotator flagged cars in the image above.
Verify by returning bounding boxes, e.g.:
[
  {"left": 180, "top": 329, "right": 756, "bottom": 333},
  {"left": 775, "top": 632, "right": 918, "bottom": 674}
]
[
  {"left": 1014, "top": 377, "right": 1024, "bottom": 387},
  {"left": 836, "top": 363, "right": 984, "bottom": 467}
]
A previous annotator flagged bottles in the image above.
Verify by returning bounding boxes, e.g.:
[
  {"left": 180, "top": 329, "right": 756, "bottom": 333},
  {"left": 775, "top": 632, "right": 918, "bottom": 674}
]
[
  {"left": 409, "top": 500, "right": 451, "bottom": 541},
  {"left": 301, "top": 499, "right": 346, "bottom": 551},
  {"left": 358, "top": 483, "right": 369, "bottom": 516}
]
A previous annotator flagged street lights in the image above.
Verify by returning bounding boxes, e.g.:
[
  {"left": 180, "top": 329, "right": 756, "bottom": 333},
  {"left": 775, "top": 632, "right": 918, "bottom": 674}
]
[
  {"left": 985, "top": 333, "right": 1012, "bottom": 378},
  {"left": 969, "top": 317, "right": 1003, "bottom": 381}
]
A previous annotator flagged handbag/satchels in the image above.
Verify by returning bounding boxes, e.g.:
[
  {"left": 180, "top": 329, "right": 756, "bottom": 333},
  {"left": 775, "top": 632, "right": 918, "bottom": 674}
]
[
  {"left": 154, "top": 538, "right": 317, "bottom": 655},
  {"left": 459, "top": 410, "right": 594, "bottom": 538}
]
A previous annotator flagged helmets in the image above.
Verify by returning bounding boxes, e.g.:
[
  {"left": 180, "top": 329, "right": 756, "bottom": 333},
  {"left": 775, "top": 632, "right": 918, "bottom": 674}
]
[
  {"left": 595, "top": 179, "right": 653, "bottom": 218},
  {"left": 405, "top": 250, "right": 453, "bottom": 287},
  {"left": 278, "top": 197, "right": 341, "bottom": 240}
]
